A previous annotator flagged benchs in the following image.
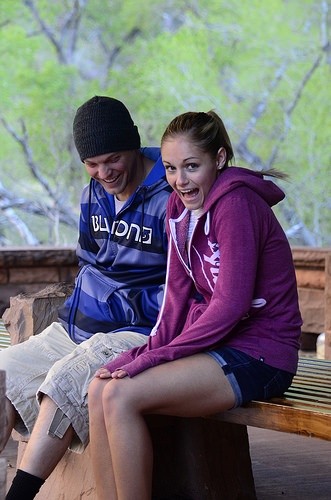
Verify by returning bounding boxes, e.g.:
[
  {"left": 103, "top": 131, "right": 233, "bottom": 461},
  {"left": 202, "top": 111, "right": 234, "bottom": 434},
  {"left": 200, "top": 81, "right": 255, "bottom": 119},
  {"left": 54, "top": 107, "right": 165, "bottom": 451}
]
[{"left": 205, "top": 356, "right": 331, "bottom": 442}]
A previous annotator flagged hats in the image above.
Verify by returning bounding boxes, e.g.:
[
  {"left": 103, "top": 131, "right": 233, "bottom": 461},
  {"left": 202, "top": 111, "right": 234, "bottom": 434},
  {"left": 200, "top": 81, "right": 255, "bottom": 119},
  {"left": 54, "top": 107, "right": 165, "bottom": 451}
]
[{"left": 73, "top": 96, "right": 140, "bottom": 162}]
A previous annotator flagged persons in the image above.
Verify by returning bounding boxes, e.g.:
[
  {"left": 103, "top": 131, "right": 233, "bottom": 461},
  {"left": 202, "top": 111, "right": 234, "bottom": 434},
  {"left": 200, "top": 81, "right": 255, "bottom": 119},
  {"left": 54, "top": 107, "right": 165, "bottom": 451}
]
[
  {"left": 86, "top": 108, "right": 304, "bottom": 500},
  {"left": 0, "top": 95, "right": 185, "bottom": 500}
]
